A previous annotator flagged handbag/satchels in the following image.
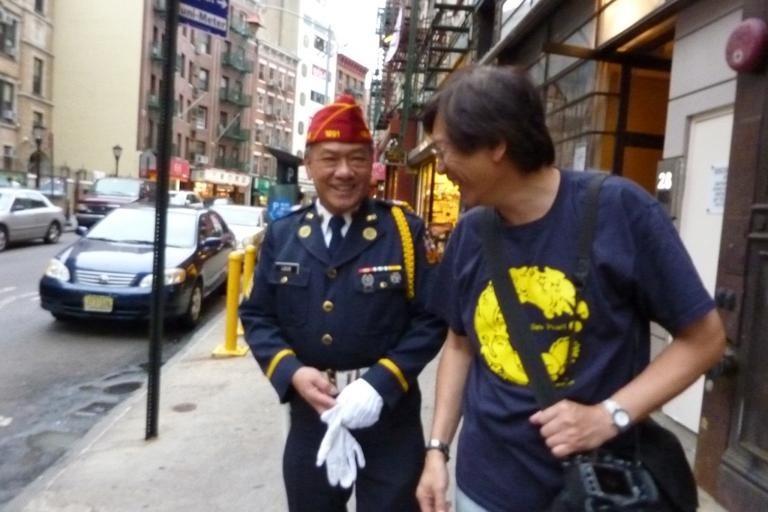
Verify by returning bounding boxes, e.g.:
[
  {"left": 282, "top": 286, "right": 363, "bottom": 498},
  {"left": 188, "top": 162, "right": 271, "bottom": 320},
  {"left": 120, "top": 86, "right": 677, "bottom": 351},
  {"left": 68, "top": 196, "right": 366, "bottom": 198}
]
[{"left": 550, "top": 415, "right": 701, "bottom": 511}]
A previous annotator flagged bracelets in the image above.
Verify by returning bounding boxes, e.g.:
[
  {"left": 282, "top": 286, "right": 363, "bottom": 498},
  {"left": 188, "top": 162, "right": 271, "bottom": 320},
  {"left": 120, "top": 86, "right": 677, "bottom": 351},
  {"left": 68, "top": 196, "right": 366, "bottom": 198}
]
[{"left": 421, "top": 437, "right": 453, "bottom": 461}]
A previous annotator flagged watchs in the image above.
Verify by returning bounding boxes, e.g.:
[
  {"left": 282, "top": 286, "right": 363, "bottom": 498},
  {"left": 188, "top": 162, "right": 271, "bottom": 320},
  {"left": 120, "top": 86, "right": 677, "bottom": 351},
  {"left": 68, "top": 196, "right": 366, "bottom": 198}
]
[{"left": 601, "top": 398, "right": 632, "bottom": 436}]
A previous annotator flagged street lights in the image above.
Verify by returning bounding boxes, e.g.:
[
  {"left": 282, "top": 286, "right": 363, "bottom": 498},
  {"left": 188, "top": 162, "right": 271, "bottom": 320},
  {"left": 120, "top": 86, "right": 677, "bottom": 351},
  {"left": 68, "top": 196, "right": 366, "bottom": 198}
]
[
  {"left": 34, "top": 122, "right": 47, "bottom": 187},
  {"left": 113, "top": 145, "right": 122, "bottom": 176}
]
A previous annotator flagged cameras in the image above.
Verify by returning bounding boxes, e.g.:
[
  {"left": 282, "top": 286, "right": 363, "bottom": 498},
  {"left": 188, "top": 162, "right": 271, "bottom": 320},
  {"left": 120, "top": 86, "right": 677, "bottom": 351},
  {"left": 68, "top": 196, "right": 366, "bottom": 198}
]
[{"left": 552, "top": 452, "right": 658, "bottom": 511}]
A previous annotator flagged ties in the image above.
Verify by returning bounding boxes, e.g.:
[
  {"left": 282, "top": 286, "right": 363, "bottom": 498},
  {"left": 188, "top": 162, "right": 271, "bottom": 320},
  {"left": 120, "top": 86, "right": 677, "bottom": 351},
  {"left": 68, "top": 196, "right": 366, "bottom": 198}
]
[{"left": 327, "top": 215, "right": 345, "bottom": 256}]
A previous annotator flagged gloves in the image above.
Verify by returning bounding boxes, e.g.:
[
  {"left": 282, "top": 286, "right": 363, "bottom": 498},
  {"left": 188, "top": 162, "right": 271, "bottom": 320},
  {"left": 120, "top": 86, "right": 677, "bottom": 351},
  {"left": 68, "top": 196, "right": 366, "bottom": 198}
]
[{"left": 314, "top": 376, "right": 385, "bottom": 489}]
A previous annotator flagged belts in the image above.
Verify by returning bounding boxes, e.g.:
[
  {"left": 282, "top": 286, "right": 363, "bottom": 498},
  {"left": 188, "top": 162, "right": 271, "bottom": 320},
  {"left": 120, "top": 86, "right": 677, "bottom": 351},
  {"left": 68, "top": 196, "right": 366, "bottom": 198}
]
[{"left": 318, "top": 367, "right": 371, "bottom": 393}]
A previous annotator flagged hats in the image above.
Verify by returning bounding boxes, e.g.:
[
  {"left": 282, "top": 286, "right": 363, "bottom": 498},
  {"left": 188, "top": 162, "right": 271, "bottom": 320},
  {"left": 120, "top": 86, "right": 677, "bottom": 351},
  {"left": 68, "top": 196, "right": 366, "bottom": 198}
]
[{"left": 304, "top": 93, "right": 373, "bottom": 144}]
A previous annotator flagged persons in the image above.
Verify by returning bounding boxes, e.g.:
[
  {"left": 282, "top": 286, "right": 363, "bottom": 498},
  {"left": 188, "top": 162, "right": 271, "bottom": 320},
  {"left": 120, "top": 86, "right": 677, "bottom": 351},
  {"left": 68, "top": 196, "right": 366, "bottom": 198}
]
[
  {"left": 413, "top": 62, "right": 727, "bottom": 512},
  {"left": 239, "top": 93, "right": 445, "bottom": 512}
]
